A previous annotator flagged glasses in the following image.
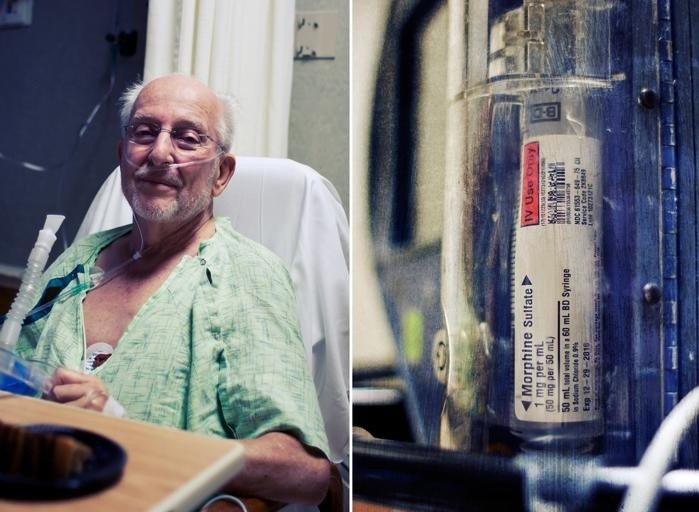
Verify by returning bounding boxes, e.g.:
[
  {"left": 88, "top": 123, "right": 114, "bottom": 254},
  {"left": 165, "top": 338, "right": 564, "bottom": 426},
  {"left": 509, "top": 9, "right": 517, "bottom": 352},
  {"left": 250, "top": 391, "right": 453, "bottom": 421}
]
[{"left": 122, "top": 122, "right": 223, "bottom": 150}]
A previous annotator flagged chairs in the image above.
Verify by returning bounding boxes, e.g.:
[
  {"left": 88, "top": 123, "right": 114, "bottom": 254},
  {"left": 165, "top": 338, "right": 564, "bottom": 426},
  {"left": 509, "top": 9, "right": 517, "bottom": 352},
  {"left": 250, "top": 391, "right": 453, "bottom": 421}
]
[{"left": 71, "top": 154, "right": 345, "bottom": 512}]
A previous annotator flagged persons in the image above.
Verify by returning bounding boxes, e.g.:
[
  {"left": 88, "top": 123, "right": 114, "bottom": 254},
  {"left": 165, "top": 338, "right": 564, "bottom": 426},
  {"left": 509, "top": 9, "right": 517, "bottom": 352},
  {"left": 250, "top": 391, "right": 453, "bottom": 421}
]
[{"left": 0, "top": 73, "right": 335, "bottom": 506}]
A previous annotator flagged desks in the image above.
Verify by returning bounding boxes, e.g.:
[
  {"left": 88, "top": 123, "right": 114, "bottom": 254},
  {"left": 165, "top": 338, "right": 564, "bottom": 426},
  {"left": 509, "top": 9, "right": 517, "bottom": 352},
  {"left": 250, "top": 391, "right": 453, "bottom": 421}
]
[{"left": 0, "top": 389, "right": 246, "bottom": 512}]
